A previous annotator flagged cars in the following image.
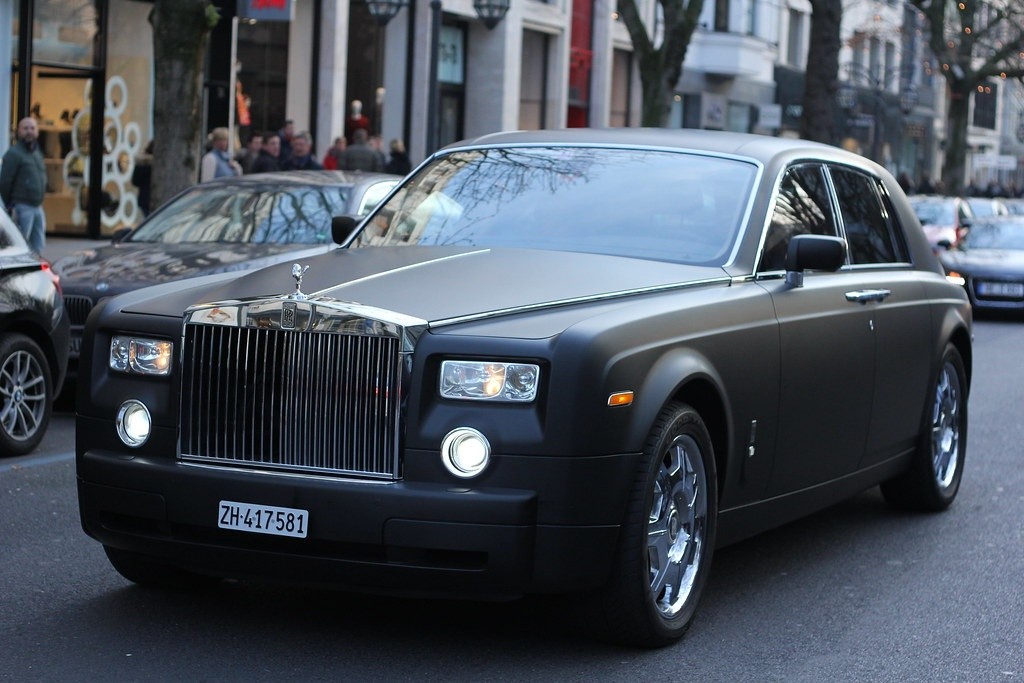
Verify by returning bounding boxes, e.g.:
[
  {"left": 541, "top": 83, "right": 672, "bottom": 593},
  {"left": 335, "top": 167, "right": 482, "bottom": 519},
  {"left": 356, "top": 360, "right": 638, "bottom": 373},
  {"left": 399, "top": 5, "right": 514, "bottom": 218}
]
[
  {"left": 72, "top": 129, "right": 973, "bottom": 651},
  {"left": 0, "top": 206, "right": 71, "bottom": 456},
  {"left": 49, "top": 171, "right": 463, "bottom": 413},
  {"left": 904, "top": 197, "right": 1024, "bottom": 313}
]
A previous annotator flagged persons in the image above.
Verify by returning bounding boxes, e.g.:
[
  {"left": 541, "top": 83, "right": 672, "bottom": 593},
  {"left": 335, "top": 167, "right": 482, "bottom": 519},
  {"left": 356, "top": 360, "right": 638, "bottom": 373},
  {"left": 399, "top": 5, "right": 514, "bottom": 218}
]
[
  {"left": 131, "top": 138, "right": 154, "bottom": 216},
  {"left": 1, "top": 117, "right": 49, "bottom": 259},
  {"left": 201, "top": 121, "right": 414, "bottom": 182},
  {"left": 371, "top": 87, "right": 385, "bottom": 136},
  {"left": 346, "top": 99, "right": 372, "bottom": 145}
]
